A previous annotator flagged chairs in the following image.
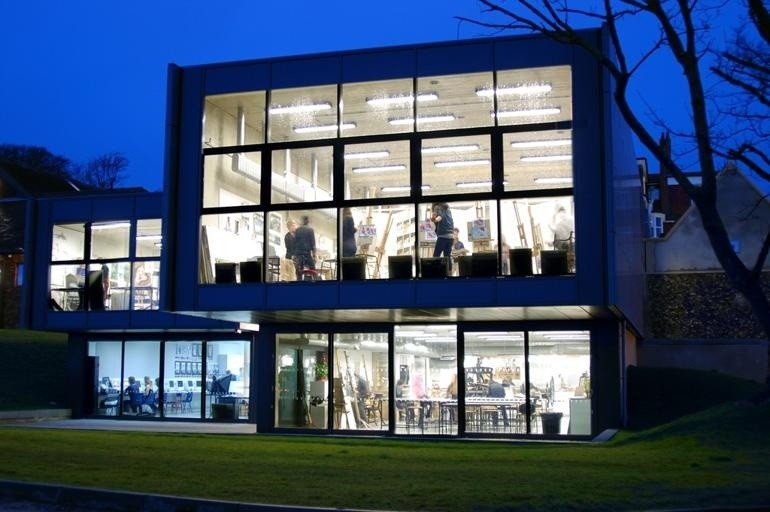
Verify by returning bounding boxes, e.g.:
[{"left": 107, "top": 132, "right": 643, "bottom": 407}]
[
  {"left": 340, "top": 255, "right": 367, "bottom": 280},
  {"left": 215, "top": 259, "right": 263, "bottom": 286},
  {"left": 104, "top": 383, "right": 194, "bottom": 416},
  {"left": 369, "top": 396, "right": 538, "bottom": 435},
  {"left": 386, "top": 246, "right": 570, "bottom": 280}
]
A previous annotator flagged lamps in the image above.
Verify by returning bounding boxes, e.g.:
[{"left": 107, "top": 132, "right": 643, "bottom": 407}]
[
  {"left": 475, "top": 81, "right": 561, "bottom": 120},
  {"left": 89, "top": 221, "right": 131, "bottom": 231},
  {"left": 380, "top": 184, "right": 431, "bottom": 193},
  {"left": 457, "top": 180, "right": 509, "bottom": 189},
  {"left": 136, "top": 233, "right": 162, "bottom": 241},
  {"left": 271, "top": 101, "right": 357, "bottom": 135},
  {"left": 344, "top": 150, "right": 406, "bottom": 174},
  {"left": 365, "top": 91, "right": 456, "bottom": 126},
  {"left": 510, "top": 138, "right": 573, "bottom": 163},
  {"left": 534, "top": 176, "right": 574, "bottom": 184},
  {"left": 421, "top": 143, "right": 491, "bottom": 170}
]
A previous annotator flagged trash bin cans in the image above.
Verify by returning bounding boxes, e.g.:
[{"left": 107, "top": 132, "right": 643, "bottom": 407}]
[{"left": 540, "top": 413, "right": 562, "bottom": 434}]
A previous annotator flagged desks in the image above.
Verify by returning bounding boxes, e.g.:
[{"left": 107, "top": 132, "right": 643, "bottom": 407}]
[{"left": 320, "top": 258, "right": 338, "bottom": 280}]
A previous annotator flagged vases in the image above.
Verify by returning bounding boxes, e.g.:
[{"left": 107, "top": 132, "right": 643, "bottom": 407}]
[{"left": 541, "top": 411, "right": 563, "bottom": 435}]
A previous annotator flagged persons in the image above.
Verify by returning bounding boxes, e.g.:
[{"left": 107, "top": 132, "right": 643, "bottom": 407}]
[
  {"left": 547, "top": 202, "right": 575, "bottom": 251},
  {"left": 431, "top": 201, "right": 455, "bottom": 276},
  {"left": 396, "top": 380, "right": 415, "bottom": 421},
  {"left": 95, "top": 256, "right": 110, "bottom": 306},
  {"left": 284, "top": 220, "right": 297, "bottom": 260},
  {"left": 494, "top": 234, "right": 512, "bottom": 276},
  {"left": 444, "top": 373, "right": 458, "bottom": 399},
  {"left": 294, "top": 215, "right": 319, "bottom": 281},
  {"left": 122, "top": 376, "right": 159, "bottom": 417},
  {"left": 412, "top": 372, "right": 433, "bottom": 418},
  {"left": 74, "top": 256, "right": 85, "bottom": 311},
  {"left": 452, "top": 227, "right": 467, "bottom": 257},
  {"left": 354, "top": 374, "right": 370, "bottom": 427},
  {"left": 342, "top": 208, "right": 357, "bottom": 256},
  {"left": 483, "top": 379, "right": 510, "bottom": 427}
]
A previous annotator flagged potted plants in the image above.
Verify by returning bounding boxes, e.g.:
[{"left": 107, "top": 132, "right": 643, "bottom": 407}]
[{"left": 314, "top": 362, "right": 328, "bottom": 382}]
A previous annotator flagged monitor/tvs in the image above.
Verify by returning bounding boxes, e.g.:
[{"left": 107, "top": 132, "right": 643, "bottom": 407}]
[{"left": 98, "top": 377, "right": 202, "bottom": 392}]
